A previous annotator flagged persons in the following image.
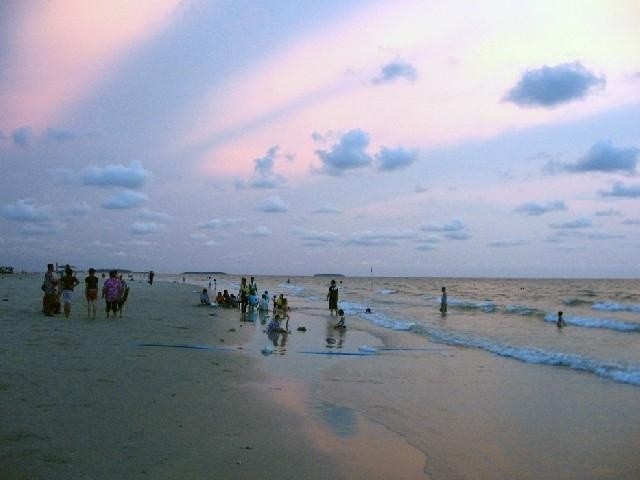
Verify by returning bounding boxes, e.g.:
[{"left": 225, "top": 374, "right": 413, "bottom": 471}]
[
  {"left": 325, "top": 279, "right": 339, "bottom": 317},
  {"left": 556, "top": 311, "right": 567, "bottom": 328},
  {"left": 438, "top": 285, "right": 448, "bottom": 315},
  {"left": 335, "top": 308, "right": 347, "bottom": 330},
  {"left": 199, "top": 276, "right": 292, "bottom": 335},
  {"left": 40, "top": 263, "right": 130, "bottom": 319},
  {"left": 148, "top": 270, "right": 155, "bottom": 285}
]
